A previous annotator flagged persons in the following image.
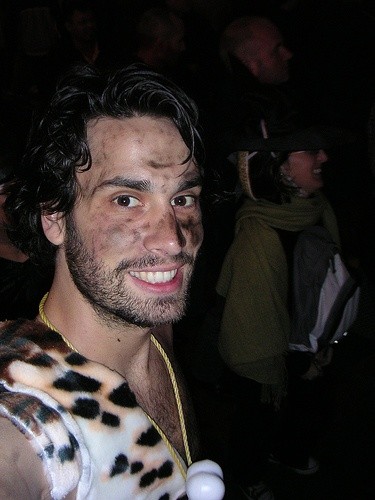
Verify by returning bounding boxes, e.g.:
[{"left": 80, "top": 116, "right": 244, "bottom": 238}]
[
  {"left": 0, "top": 1, "right": 357, "bottom": 499},
  {"left": 0, "top": 65, "right": 206, "bottom": 500}
]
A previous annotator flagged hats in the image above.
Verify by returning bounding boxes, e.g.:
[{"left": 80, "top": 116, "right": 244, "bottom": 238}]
[{"left": 234, "top": 115, "right": 326, "bottom": 152}]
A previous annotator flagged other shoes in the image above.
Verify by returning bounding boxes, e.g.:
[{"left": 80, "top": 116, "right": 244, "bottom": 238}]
[
  {"left": 268, "top": 453, "right": 320, "bottom": 474},
  {"left": 239, "top": 480, "right": 274, "bottom": 500}
]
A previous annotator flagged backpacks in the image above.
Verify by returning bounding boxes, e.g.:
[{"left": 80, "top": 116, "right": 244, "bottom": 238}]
[{"left": 288, "top": 224, "right": 361, "bottom": 352}]
